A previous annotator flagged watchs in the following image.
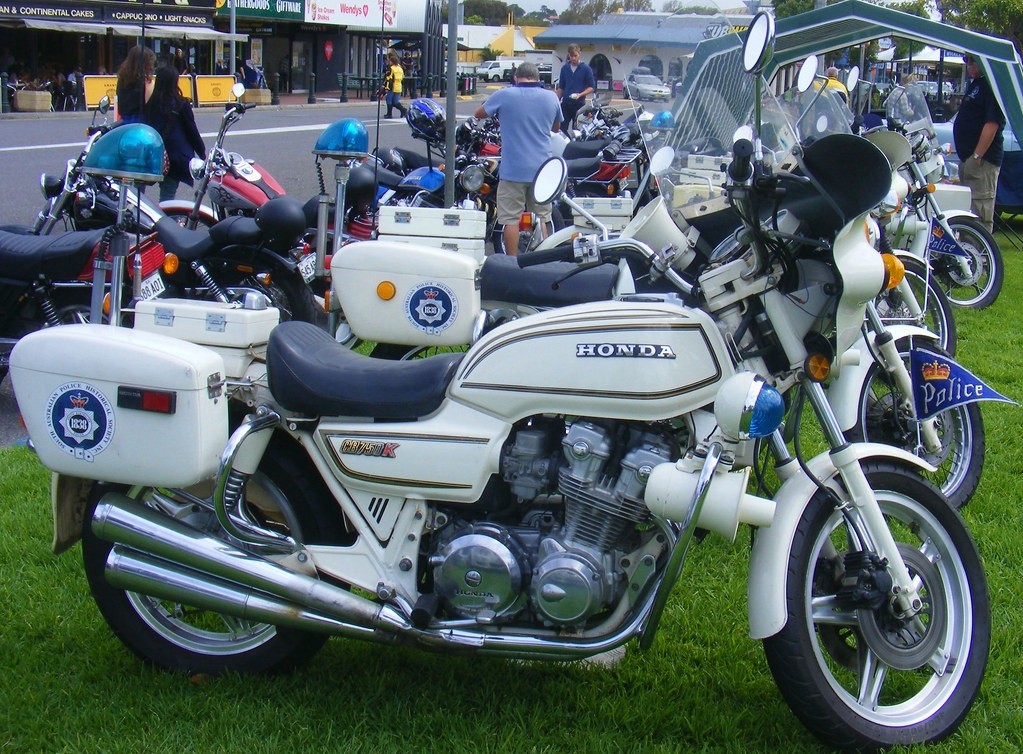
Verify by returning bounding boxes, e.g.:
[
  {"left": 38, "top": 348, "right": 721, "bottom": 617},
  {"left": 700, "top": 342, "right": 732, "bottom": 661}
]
[{"left": 972, "top": 152, "right": 982, "bottom": 161}]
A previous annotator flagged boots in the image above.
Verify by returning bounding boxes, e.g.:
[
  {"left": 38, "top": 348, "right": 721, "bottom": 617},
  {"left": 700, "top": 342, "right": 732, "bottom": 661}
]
[
  {"left": 396, "top": 103, "right": 407, "bottom": 118},
  {"left": 384, "top": 105, "right": 392, "bottom": 118}
]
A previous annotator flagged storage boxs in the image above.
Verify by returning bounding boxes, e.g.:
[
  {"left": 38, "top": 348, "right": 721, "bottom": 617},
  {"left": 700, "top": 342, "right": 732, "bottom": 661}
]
[
  {"left": 378, "top": 205, "right": 488, "bottom": 264},
  {"left": 133, "top": 296, "right": 282, "bottom": 382},
  {"left": 677, "top": 153, "right": 731, "bottom": 186},
  {"left": 8, "top": 324, "right": 229, "bottom": 489},
  {"left": 571, "top": 196, "right": 633, "bottom": 231},
  {"left": 331, "top": 240, "right": 481, "bottom": 345}
]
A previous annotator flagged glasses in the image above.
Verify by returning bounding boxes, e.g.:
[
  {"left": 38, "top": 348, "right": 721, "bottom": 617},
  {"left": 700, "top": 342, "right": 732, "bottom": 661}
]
[{"left": 963, "top": 55, "right": 976, "bottom": 63}]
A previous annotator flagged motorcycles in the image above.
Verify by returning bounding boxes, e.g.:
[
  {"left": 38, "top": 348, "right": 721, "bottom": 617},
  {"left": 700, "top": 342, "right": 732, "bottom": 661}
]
[{"left": 0, "top": 0, "right": 1007, "bottom": 754}]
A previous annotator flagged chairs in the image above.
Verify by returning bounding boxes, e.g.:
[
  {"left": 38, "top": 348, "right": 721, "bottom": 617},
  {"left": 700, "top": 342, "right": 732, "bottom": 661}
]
[
  {"left": 7, "top": 78, "right": 79, "bottom": 112},
  {"left": 993, "top": 149, "right": 1023, "bottom": 252}
]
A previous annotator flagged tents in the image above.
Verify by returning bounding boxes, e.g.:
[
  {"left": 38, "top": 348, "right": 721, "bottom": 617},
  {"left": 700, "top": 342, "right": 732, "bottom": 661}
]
[{"left": 661, "top": 0, "right": 1023, "bottom": 184}]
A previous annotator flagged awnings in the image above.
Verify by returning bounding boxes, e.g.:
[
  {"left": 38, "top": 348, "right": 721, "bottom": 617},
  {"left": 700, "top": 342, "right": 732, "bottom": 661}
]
[{"left": 23, "top": 18, "right": 249, "bottom": 42}]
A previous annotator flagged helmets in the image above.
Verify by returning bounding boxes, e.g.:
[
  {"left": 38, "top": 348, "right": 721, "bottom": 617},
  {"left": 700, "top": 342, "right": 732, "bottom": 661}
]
[{"left": 407, "top": 98, "right": 446, "bottom": 140}]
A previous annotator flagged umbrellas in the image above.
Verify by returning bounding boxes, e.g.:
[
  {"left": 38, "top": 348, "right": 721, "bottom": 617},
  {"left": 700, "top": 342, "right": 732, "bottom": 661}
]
[{"left": 388, "top": 35, "right": 470, "bottom": 51}]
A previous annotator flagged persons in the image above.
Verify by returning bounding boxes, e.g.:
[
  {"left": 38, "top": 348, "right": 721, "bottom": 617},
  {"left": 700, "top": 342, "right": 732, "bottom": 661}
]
[
  {"left": 475, "top": 62, "right": 564, "bottom": 255},
  {"left": 279, "top": 54, "right": 289, "bottom": 89},
  {"left": 174, "top": 48, "right": 188, "bottom": 75},
  {"left": 823, "top": 67, "right": 848, "bottom": 108},
  {"left": 883, "top": 73, "right": 919, "bottom": 125},
  {"left": 144, "top": 65, "right": 206, "bottom": 223},
  {"left": 116, "top": 45, "right": 157, "bottom": 124},
  {"left": 557, "top": 43, "right": 595, "bottom": 140},
  {"left": 401, "top": 52, "right": 413, "bottom": 76},
  {"left": 512, "top": 63, "right": 517, "bottom": 86},
  {"left": 456, "top": 72, "right": 465, "bottom": 78},
  {"left": 953, "top": 51, "right": 1006, "bottom": 262},
  {"left": 383, "top": 53, "right": 407, "bottom": 118},
  {"left": 0, "top": 47, "right": 109, "bottom": 95},
  {"left": 227, "top": 58, "right": 257, "bottom": 82}
]
